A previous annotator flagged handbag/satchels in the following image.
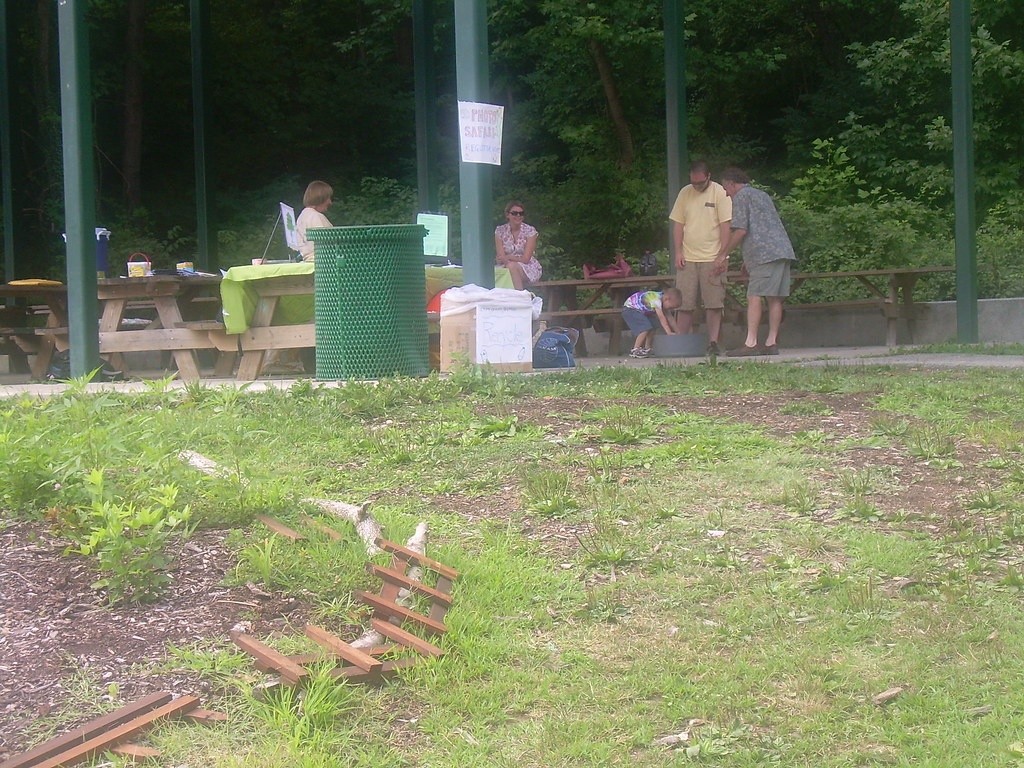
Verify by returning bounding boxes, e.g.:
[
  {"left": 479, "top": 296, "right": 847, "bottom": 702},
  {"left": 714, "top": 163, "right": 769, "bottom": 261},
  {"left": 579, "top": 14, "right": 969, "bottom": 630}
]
[{"left": 533, "top": 326, "right": 580, "bottom": 368}]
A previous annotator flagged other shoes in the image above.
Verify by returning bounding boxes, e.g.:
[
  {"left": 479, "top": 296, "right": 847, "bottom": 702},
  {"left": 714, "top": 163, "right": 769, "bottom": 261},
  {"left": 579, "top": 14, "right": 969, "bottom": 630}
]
[
  {"left": 726, "top": 344, "right": 759, "bottom": 356},
  {"left": 742, "top": 344, "right": 779, "bottom": 355},
  {"left": 706, "top": 341, "right": 720, "bottom": 355}
]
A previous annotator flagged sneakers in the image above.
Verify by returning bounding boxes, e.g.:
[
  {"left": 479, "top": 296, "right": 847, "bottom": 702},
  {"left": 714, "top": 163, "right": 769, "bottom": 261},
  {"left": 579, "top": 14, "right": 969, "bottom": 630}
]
[
  {"left": 643, "top": 348, "right": 656, "bottom": 357},
  {"left": 629, "top": 347, "right": 647, "bottom": 358}
]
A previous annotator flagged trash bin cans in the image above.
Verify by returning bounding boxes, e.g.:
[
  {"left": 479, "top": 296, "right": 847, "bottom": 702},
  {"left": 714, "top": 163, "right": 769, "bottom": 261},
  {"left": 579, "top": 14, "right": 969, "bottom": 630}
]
[{"left": 306, "top": 223, "right": 430, "bottom": 381}]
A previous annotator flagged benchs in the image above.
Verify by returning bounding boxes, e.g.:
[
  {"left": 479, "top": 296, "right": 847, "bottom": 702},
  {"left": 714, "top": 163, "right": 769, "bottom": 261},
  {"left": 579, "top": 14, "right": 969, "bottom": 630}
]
[
  {"left": 34, "top": 327, "right": 68, "bottom": 337},
  {"left": 732, "top": 298, "right": 936, "bottom": 325},
  {"left": 174, "top": 319, "right": 225, "bottom": 332},
  {"left": 538, "top": 307, "right": 623, "bottom": 320}
]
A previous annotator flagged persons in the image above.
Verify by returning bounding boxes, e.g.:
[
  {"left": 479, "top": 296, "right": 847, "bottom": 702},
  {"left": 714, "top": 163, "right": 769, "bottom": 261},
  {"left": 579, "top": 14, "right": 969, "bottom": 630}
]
[
  {"left": 622, "top": 287, "right": 683, "bottom": 358},
  {"left": 297, "top": 180, "right": 333, "bottom": 262},
  {"left": 668, "top": 161, "right": 733, "bottom": 355},
  {"left": 714, "top": 166, "right": 796, "bottom": 356},
  {"left": 495, "top": 199, "right": 543, "bottom": 290}
]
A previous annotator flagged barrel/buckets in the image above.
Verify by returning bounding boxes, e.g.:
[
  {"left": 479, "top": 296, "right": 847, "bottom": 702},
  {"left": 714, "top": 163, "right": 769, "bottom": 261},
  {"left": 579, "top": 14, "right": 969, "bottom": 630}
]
[{"left": 126, "top": 253, "right": 151, "bottom": 278}]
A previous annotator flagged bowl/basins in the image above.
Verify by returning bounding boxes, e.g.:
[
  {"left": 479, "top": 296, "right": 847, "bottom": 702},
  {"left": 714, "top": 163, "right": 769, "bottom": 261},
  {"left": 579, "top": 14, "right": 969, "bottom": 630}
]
[{"left": 651, "top": 333, "right": 708, "bottom": 358}]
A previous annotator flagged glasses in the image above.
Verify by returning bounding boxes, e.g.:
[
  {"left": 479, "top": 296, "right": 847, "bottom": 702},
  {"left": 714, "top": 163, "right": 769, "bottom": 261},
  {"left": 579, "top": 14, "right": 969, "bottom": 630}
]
[{"left": 508, "top": 211, "right": 524, "bottom": 216}]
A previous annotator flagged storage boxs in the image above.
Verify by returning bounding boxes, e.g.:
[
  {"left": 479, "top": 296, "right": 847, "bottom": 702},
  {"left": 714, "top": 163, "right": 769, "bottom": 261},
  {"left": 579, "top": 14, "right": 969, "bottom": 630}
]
[{"left": 440, "top": 295, "right": 534, "bottom": 373}]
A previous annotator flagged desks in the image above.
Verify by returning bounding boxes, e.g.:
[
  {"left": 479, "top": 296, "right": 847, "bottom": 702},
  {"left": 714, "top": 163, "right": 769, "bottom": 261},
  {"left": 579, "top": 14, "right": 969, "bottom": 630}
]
[
  {"left": 532, "top": 275, "right": 676, "bottom": 357},
  {"left": 724, "top": 267, "right": 957, "bottom": 346},
  {"left": 0, "top": 262, "right": 514, "bottom": 388}
]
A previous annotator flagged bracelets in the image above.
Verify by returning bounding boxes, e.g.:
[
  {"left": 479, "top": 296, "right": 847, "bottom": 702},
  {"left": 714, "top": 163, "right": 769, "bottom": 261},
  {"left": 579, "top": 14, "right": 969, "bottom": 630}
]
[{"left": 726, "top": 255, "right": 730, "bottom": 260}]
